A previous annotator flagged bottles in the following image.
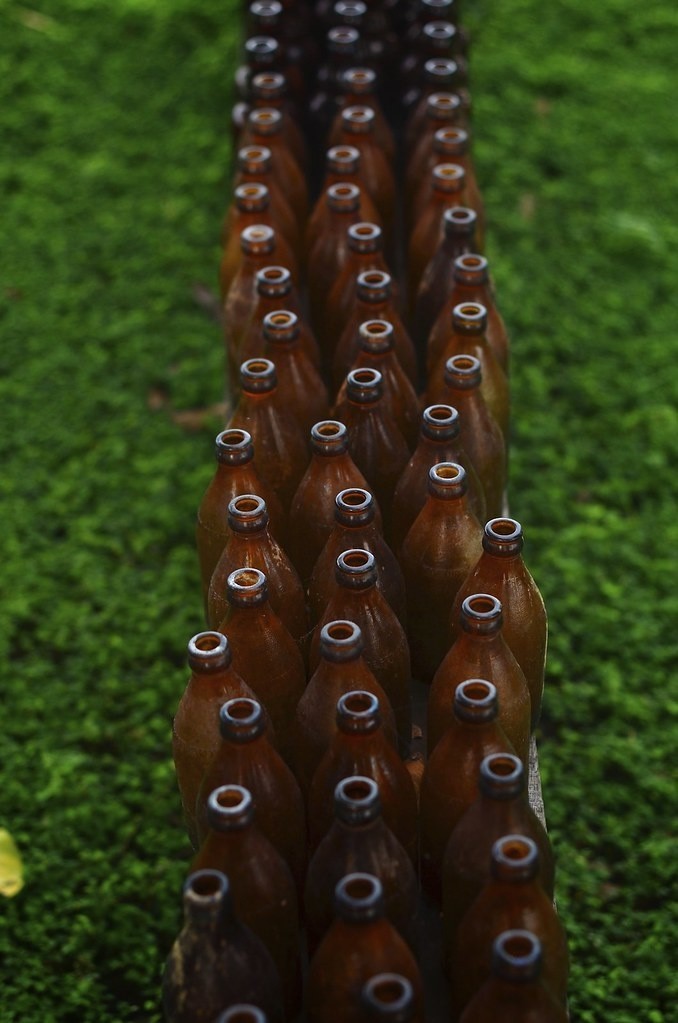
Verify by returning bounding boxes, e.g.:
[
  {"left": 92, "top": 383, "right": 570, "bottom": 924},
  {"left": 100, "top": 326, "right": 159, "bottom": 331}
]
[{"left": 170, "top": 0, "right": 568, "bottom": 1023}]
